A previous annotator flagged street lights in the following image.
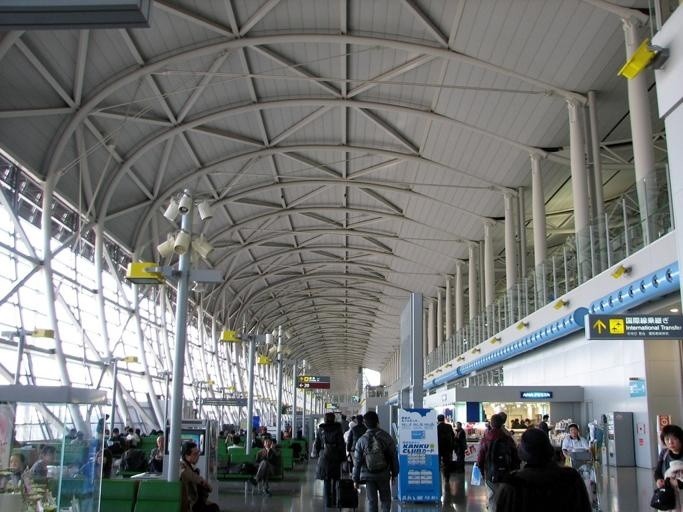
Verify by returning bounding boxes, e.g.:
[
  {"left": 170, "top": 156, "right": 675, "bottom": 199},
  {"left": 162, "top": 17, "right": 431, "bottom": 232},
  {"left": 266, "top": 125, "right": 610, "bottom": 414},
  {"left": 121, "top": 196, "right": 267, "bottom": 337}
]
[{"left": 0, "top": 324, "right": 140, "bottom": 442}]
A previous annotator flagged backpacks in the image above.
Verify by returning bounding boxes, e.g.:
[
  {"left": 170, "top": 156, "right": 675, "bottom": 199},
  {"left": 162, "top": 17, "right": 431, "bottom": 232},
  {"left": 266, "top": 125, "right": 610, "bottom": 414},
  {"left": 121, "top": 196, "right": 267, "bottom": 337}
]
[
  {"left": 485, "top": 433, "right": 520, "bottom": 483},
  {"left": 362, "top": 430, "right": 393, "bottom": 474}
]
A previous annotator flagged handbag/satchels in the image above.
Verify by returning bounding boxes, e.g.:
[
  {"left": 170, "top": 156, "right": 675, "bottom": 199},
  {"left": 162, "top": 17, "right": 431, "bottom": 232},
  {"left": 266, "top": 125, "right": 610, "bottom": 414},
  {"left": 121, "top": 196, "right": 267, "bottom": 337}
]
[
  {"left": 470, "top": 461, "right": 485, "bottom": 487},
  {"left": 650, "top": 479, "right": 676, "bottom": 511}
]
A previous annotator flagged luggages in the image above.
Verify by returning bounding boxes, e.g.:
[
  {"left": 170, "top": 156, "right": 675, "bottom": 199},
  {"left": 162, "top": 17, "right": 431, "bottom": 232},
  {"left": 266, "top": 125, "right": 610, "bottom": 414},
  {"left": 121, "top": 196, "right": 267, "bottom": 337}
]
[{"left": 335, "top": 460, "right": 359, "bottom": 512}]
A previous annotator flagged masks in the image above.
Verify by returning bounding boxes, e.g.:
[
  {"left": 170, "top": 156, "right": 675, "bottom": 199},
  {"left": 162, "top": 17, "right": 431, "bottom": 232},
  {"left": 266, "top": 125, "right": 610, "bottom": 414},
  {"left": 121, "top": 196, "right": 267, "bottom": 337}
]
[{"left": 97, "top": 456, "right": 108, "bottom": 464}]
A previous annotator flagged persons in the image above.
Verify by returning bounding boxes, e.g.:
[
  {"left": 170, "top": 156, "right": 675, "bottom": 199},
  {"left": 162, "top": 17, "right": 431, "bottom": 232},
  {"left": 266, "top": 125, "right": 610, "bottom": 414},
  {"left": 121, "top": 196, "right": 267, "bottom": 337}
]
[
  {"left": 0, "top": 409, "right": 601, "bottom": 493},
  {"left": 350, "top": 410, "right": 398, "bottom": 511},
  {"left": 174, "top": 440, "right": 219, "bottom": 511},
  {"left": 654, "top": 424, "right": 683, "bottom": 511},
  {"left": 488, "top": 428, "right": 593, "bottom": 512},
  {"left": 309, "top": 412, "right": 348, "bottom": 508}
]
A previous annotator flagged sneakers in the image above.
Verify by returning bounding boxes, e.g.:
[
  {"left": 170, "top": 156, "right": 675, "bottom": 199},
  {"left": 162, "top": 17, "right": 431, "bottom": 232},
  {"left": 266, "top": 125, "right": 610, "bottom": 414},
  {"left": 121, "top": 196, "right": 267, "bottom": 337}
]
[
  {"left": 323, "top": 496, "right": 332, "bottom": 508},
  {"left": 247, "top": 477, "right": 258, "bottom": 488},
  {"left": 262, "top": 489, "right": 272, "bottom": 497}
]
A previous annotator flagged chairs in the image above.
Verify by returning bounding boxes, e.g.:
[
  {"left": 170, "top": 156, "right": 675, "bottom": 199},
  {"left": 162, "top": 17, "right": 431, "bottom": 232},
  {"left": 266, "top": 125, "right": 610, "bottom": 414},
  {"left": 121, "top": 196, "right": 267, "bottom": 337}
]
[{"left": 13, "top": 433, "right": 308, "bottom": 511}]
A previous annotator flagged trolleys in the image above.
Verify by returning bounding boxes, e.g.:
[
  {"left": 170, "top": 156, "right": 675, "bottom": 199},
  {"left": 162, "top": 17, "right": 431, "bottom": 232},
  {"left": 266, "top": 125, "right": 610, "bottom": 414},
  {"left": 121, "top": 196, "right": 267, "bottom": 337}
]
[{"left": 565, "top": 447, "right": 603, "bottom": 512}]
